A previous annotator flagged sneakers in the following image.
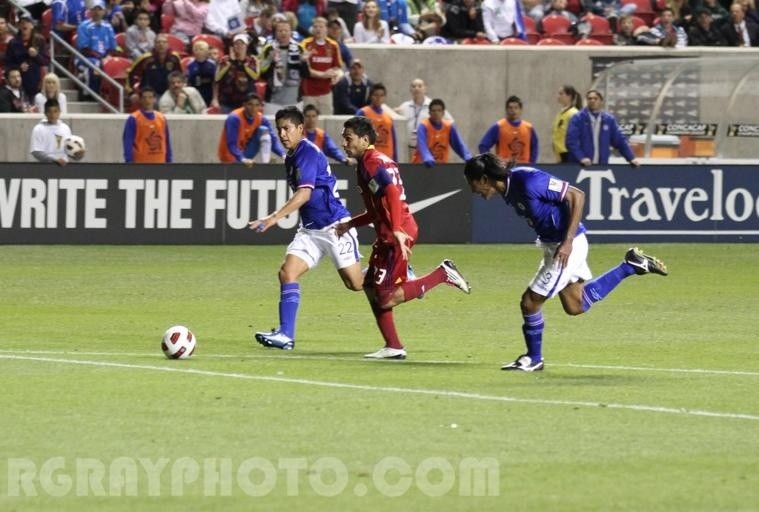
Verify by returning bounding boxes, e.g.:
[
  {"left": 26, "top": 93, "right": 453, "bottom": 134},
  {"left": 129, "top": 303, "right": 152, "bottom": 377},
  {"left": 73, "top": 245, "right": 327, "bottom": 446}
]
[
  {"left": 255, "top": 327, "right": 294, "bottom": 350},
  {"left": 623, "top": 247, "right": 668, "bottom": 276},
  {"left": 437, "top": 259, "right": 471, "bottom": 294},
  {"left": 501, "top": 355, "right": 545, "bottom": 372},
  {"left": 406, "top": 264, "right": 424, "bottom": 299},
  {"left": 363, "top": 346, "right": 405, "bottom": 359}
]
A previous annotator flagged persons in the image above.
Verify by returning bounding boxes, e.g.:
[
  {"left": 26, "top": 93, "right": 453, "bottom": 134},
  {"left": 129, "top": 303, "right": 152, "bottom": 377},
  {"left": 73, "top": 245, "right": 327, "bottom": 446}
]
[
  {"left": 218, "top": 92, "right": 286, "bottom": 163},
  {"left": 332, "top": 116, "right": 471, "bottom": 360},
  {"left": 301, "top": 103, "right": 353, "bottom": 166},
  {"left": 565, "top": 90, "right": 641, "bottom": 166},
  {"left": 392, "top": 78, "right": 453, "bottom": 164},
  {"left": 247, "top": 107, "right": 424, "bottom": 351},
  {"left": 551, "top": 83, "right": 583, "bottom": 164},
  {"left": 390, "top": 0, "right": 758, "bottom": 46},
  {"left": 122, "top": 88, "right": 172, "bottom": 163},
  {"left": 477, "top": 95, "right": 539, "bottom": 164},
  {"left": 29, "top": 100, "right": 86, "bottom": 164},
  {"left": 1, "top": 0, "right": 390, "bottom": 113},
  {"left": 417, "top": 99, "right": 472, "bottom": 167},
  {"left": 464, "top": 151, "right": 668, "bottom": 372},
  {"left": 356, "top": 81, "right": 399, "bottom": 163}
]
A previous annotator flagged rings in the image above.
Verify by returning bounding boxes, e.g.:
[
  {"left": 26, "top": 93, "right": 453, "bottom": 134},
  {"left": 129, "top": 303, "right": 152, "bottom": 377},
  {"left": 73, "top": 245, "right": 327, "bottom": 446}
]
[{"left": 258, "top": 224, "right": 265, "bottom": 229}]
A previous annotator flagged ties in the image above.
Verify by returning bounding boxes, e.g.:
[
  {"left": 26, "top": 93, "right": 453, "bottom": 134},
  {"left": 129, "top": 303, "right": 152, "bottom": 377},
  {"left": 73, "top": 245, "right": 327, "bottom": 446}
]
[{"left": 739, "top": 26, "right": 744, "bottom": 43}]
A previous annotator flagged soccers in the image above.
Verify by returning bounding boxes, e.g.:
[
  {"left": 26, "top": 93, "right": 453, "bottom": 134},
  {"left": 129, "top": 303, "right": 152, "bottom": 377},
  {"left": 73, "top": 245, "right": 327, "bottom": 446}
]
[
  {"left": 161, "top": 325, "right": 198, "bottom": 359},
  {"left": 63, "top": 135, "right": 84, "bottom": 158}
]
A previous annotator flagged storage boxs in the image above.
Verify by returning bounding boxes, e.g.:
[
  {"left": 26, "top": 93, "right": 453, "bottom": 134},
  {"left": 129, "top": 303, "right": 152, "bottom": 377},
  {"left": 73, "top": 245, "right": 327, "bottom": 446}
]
[{"left": 629, "top": 134, "right": 680, "bottom": 159}]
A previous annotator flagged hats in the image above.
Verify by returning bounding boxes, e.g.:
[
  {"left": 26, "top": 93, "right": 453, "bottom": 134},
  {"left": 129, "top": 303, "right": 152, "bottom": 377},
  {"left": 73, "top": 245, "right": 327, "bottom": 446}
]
[
  {"left": 694, "top": 6, "right": 710, "bottom": 18},
  {"left": 90, "top": 1, "right": 107, "bottom": 11},
  {"left": 19, "top": 13, "right": 33, "bottom": 24},
  {"left": 233, "top": 33, "right": 248, "bottom": 45},
  {"left": 350, "top": 58, "right": 363, "bottom": 69}
]
